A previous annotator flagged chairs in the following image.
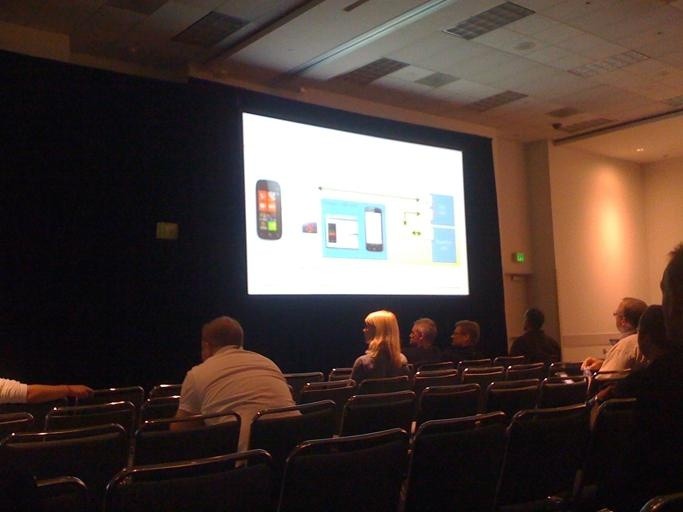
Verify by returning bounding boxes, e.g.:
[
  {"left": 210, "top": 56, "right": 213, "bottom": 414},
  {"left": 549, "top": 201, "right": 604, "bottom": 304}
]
[{"left": 0, "top": 356, "right": 683, "bottom": 512}]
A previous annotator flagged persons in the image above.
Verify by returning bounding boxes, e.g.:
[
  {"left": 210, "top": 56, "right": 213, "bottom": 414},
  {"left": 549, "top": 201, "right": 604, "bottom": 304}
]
[
  {"left": 403, "top": 318, "right": 442, "bottom": 366},
  {"left": 348, "top": 310, "right": 408, "bottom": 383},
  {"left": 443, "top": 320, "right": 487, "bottom": 362},
  {"left": 169, "top": 314, "right": 300, "bottom": 469},
  {"left": 580, "top": 243, "right": 683, "bottom": 511},
  {"left": 0, "top": 376, "right": 93, "bottom": 406},
  {"left": 508, "top": 309, "right": 560, "bottom": 363}
]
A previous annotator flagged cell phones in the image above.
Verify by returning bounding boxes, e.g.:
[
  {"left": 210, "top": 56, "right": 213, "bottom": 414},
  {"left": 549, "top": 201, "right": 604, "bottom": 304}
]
[
  {"left": 365, "top": 208, "right": 383, "bottom": 251},
  {"left": 256, "top": 180, "right": 281, "bottom": 240},
  {"left": 328, "top": 224, "right": 336, "bottom": 243}
]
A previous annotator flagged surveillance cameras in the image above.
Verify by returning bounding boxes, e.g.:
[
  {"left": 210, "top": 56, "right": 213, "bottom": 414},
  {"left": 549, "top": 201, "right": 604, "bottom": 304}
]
[{"left": 552, "top": 123, "right": 561, "bottom": 128}]
